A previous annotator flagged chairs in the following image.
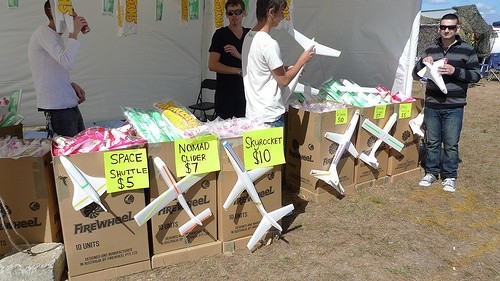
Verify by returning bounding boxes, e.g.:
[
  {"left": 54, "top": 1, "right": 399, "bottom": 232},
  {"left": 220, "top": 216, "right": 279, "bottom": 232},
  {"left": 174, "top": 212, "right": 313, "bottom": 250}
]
[{"left": 188, "top": 78, "right": 217, "bottom": 122}]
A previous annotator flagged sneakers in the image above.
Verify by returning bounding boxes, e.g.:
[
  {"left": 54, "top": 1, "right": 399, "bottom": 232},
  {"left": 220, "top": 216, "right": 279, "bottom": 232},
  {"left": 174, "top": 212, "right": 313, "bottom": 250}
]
[
  {"left": 418, "top": 172, "right": 437, "bottom": 187},
  {"left": 441, "top": 178, "right": 456, "bottom": 192}
]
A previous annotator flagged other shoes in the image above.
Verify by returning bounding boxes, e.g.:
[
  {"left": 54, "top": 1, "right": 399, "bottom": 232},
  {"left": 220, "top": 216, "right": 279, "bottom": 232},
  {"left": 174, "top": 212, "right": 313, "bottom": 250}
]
[{"left": 285, "top": 211, "right": 293, "bottom": 216}]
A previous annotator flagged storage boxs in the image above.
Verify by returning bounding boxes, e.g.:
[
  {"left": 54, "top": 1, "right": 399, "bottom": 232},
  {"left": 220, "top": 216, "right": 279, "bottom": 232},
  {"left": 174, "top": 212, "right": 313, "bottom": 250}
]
[{"left": 0, "top": 97, "right": 424, "bottom": 281}]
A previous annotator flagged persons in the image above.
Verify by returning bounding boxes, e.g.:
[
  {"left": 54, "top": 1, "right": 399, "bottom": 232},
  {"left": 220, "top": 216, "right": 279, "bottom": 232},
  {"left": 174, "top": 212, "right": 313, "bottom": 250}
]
[
  {"left": 241, "top": 0, "right": 314, "bottom": 216},
  {"left": 207, "top": 0, "right": 252, "bottom": 117},
  {"left": 412, "top": 13, "right": 481, "bottom": 192},
  {"left": 29, "top": 0, "right": 88, "bottom": 137}
]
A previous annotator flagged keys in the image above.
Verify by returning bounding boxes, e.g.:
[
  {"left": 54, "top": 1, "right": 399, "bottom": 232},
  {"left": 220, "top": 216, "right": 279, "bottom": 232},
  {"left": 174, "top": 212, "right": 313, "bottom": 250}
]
[{"left": 43, "top": 110, "right": 53, "bottom": 138}]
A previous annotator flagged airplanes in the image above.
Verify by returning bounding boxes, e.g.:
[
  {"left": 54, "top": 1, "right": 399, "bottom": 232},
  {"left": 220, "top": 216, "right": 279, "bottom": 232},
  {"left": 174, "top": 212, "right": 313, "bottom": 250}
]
[
  {"left": 416, "top": 57, "right": 448, "bottom": 95},
  {"left": 279, "top": 24, "right": 341, "bottom": 104},
  {"left": 310, "top": 109, "right": 361, "bottom": 196},
  {"left": 222, "top": 141, "right": 295, "bottom": 251},
  {"left": 134, "top": 156, "right": 212, "bottom": 237},
  {"left": 336, "top": 78, "right": 381, "bottom": 107},
  {"left": 59, "top": 155, "right": 109, "bottom": 213},
  {"left": 358, "top": 113, "right": 405, "bottom": 169}
]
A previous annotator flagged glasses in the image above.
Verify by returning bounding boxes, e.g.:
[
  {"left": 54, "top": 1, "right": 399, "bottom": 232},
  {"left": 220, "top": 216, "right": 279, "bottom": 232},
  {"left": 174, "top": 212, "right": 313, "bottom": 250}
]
[
  {"left": 226, "top": 8, "right": 244, "bottom": 16},
  {"left": 439, "top": 25, "right": 457, "bottom": 30}
]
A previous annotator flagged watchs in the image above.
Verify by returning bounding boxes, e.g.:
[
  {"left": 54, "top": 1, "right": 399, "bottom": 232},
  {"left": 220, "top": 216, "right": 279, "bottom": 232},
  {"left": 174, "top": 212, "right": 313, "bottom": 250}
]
[{"left": 240, "top": 68, "right": 242, "bottom": 75}]
